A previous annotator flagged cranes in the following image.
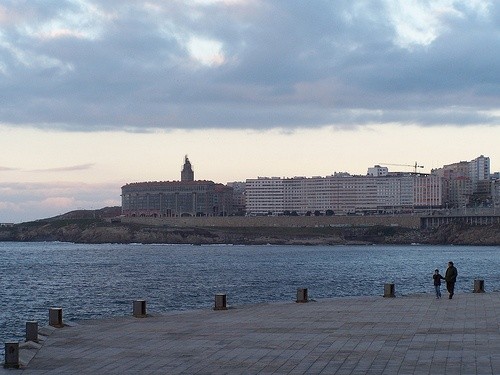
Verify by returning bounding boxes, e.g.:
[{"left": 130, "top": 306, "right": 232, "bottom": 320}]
[{"left": 381, "top": 161, "right": 424, "bottom": 173}]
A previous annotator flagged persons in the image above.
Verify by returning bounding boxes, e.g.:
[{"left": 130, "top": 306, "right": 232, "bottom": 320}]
[{"left": 433, "top": 261, "right": 457, "bottom": 299}]
